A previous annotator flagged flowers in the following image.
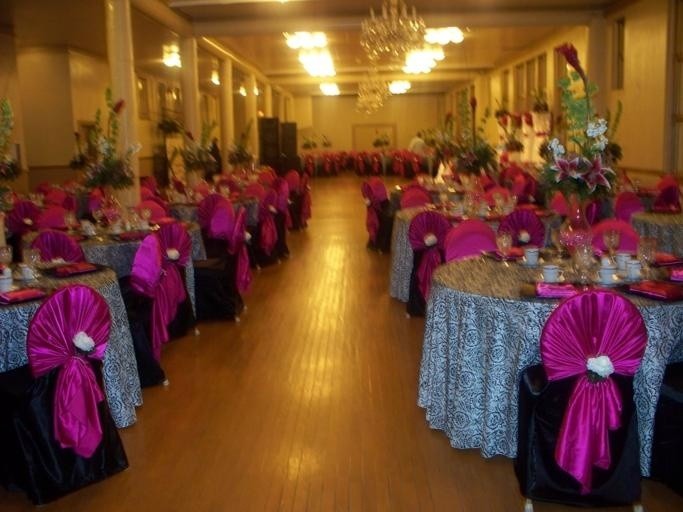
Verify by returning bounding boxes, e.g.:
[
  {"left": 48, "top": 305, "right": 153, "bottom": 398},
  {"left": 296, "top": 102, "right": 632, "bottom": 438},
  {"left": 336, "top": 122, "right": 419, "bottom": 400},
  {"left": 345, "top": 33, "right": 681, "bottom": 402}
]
[
  {"left": 227, "top": 117, "right": 255, "bottom": 186},
  {"left": 418, "top": 79, "right": 503, "bottom": 176},
  {"left": 65, "top": 85, "right": 143, "bottom": 191},
  {"left": 159, "top": 116, "right": 218, "bottom": 173},
  {"left": 537, "top": 41, "right": 645, "bottom": 202}
]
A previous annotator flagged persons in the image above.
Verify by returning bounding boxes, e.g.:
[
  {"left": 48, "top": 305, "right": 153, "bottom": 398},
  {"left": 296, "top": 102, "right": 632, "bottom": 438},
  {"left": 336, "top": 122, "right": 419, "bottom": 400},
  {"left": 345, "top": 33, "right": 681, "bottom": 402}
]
[
  {"left": 210, "top": 136, "right": 219, "bottom": 159},
  {"left": 407, "top": 132, "right": 426, "bottom": 155}
]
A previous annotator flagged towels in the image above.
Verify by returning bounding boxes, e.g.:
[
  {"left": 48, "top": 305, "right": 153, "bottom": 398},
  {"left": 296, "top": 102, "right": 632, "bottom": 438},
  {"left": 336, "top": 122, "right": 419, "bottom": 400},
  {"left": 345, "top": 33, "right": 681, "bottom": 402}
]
[
  {"left": 536, "top": 281, "right": 579, "bottom": 298},
  {"left": 670, "top": 268, "right": 683, "bottom": 281},
  {"left": 56, "top": 264, "right": 96, "bottom": 275},
  {"left": 629, "top": 281, "right": 683, "bottom": 301},
  {"left": 1, "top": 289, "right": 44, "bottom": 303},
  {"left": 157, "top": 217, "right": 175, "bottom": 225},
  {"left": 121, "top": 231, "right": 144, "bottom": 240},
  {"left": 654, "top": 253, "right": 682, "bottom": 267},
  {"left": 497, "top": 247, "right": 523, "bottom": 258}
]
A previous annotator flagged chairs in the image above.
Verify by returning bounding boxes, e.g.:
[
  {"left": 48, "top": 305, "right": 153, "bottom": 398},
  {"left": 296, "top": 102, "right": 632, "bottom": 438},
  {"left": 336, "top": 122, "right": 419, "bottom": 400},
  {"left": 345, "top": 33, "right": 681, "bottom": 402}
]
[
  {"left": 192, "top": 206, "right": 252, "bottom": 322},
  {"left": 28, "top": 230, "right": 89, "bottom": 269},
  {"left": 516, "top": 289, "right": 649, "bottom": 512},
  {"left": 4, "top": 199, "right": 43, "bottom": 263},
  {"left": 0, "top": 284, "right": 129, "bottom": 512},
  {"left": 500, "top": 166, "right": 538, "bottom": 204},
  {"left": 196, "top": 193, "right": 235, "bottom": 253},
  {"left": 496, "top": 209, "right": 546, "bottom": 252},
  {"left": 34, "top": 207, "right": 77, "bottom": 232},
  {"left": 274, "top": 171, "right": 301, "bottom": 230},
  {"left": 588, "top": 218, "right": 642, "bottom": 262},
  {"left": 479, "top": 187, "right": 511, "bottom": 206},
  {"left": 140, "top": 176, "right": 166, "bottom": 206},
  {"left": 614, "top": 192, "right": 644, "bottom": 225},
  {"left": 400, "top": 187, "right": 432, "bottom": 211},
  {"left": 406, "top": 212, "right": 454, "bottom": 316},
  {"left": 303, "top": 148, "right": 424, "bottom": 178},
  {"left": 33, "top": 180, "right": 102, "bottom": 216},
  {"left": 298, "top": 174, "right": 312, "bottom": 227},
  {"left": 360, "top": 175, "right": 398, "bottom": 252},
  {"left": 134, "top": 201, "right": 170, "bottom": 226},
  {"left": 443, "top": 220, "right": 497, "bottom": 263},
  {"left": 651, "top": 175, "right": 683, "bottom": 215},
  {"left": 153, "top": 223, "right": 198, "bottom": 340},
  {"left": 115, "top": 233, "right": 170, "bottom": 389},
  {"left": 244, "top": 178, "right": 288, "bottom": 269}
]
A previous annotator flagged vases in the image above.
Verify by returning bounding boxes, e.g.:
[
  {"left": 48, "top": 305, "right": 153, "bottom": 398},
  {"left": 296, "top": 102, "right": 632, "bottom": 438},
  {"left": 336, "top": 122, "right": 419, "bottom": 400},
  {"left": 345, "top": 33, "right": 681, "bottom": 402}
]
[
  {"left": 99, "top": 183, "right": 125, "bottom": 232},
  {"left": 231, "top": 157, "right": 250, "bottom": 186},
  {"left": 185, "top": 165, "right": 207, "bottom": 205},
  {"left": 458, "top": 169, "right": 487, "bottom": 219},
  {"left": 559, "top": 190, "right": 595, "bottom": 273}
]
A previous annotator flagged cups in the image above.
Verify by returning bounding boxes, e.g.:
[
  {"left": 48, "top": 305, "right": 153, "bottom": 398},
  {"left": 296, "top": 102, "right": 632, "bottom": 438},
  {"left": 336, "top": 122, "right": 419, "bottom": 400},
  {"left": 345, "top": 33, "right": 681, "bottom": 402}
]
[
  {"left": 601, "top": 257, "right": 612, "bottom": 268},
  {"left": 625, "top": 260, "right": 641, "bottom": 282},
  {"left": 596, "top": 269, "right": 617, "bottom": 288},
  {"left": 1, "top": 275, "right": 13, "bottom": 293},
  {"left": 91, "top": 207, "right": 104, "bottom": 224},
  {"left": 496, "top": 231, "right": 513, "bottom": 269},
  {"left": 141, "top": 207, "right": 152, "bottom": 221},
  {"left": 65, "top": 212, "right": 77, "bottom": 234},
  {"left": 109, "top": 223, "right": 120, "bottom": 232},
  {"left": 0, "top": 247, "right": 14, "bottom": 267},
  {"left": 23, "top": 248, "right": 39, "bottom": 272},
  {"left": 542, "top": 265, "right": 564, "bottom": 283},
  {"left": 574, "top": 243, "right": 594, "bottom": 289},
  {"left": 636, "top": 235, "right": 657, "bottom": 280},
  {"left": 603, "top": 230, "right": 620, "bottom": 256},
  {"left": 613, "top": 253, "right": 631, "bottom": 280},
  {"left": 523, "top": 248, "right": 540, "bottom": 268}
]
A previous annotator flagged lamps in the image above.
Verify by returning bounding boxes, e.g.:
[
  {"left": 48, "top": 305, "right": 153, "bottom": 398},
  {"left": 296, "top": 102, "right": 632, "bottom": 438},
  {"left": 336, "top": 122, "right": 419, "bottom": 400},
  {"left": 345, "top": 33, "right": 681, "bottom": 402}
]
[
  {"left": 358, "top": 0, "right": 428, "bottom": 73},
  {"left": 353, "top": 81, "right": 392, "bottom": 115}
]
